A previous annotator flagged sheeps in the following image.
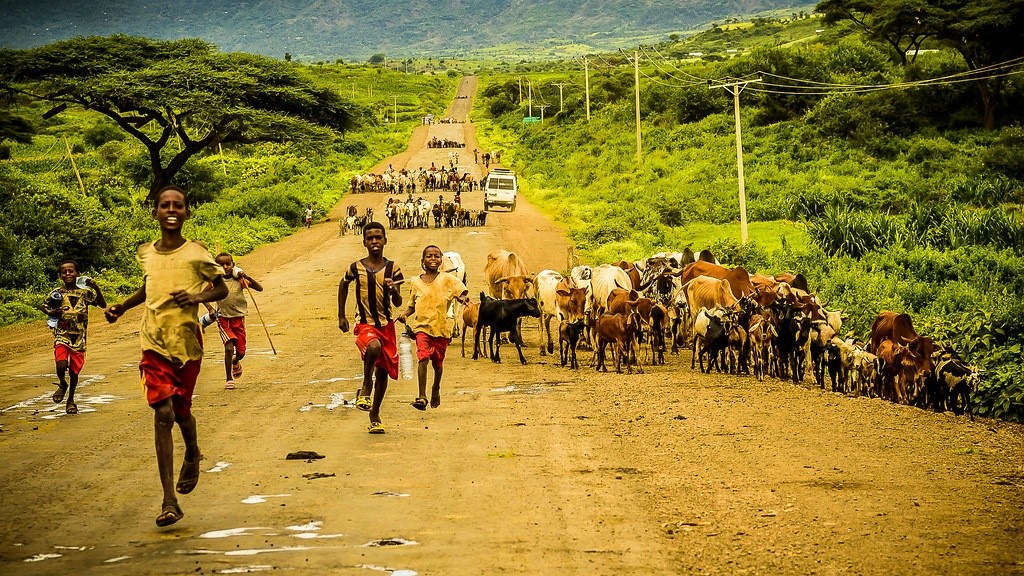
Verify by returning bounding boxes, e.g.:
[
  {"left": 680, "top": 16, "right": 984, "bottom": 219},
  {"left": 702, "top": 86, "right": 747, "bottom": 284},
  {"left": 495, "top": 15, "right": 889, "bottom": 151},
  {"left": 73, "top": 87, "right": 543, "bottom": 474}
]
[
  {"left": 427, "top": 138, "right": 466, "bottom": 148},
  {"left": 347, "top": 167, "right": 488, "bottom": 194},
  {"left": 339, "top": 205, "right": 374, "bottom": 235},
  {"left": 384, "top": 195, "right": 489, "bottom": 231},
  {"left": 438, "top": 246, "right": 981, "bottom": 424},
  {"left": 440, "top": 118, "right": 466, "bottom": 123}
]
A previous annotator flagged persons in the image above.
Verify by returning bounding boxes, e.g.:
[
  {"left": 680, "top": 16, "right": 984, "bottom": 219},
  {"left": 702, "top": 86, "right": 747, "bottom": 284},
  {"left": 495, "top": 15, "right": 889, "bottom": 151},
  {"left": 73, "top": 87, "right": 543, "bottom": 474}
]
[
  {"left": 453, "top": 152, "right": 459, "bottom": 164},
  {"left": 338, "top": 222, "right": 405, "bottom": 434},
  {"left": 203, "top": 252, "right": 263, "bottom": 388},
  {"left": 387, "top": 163, "right": 393, "bottom": 173},
  {"left": 40, "top": 261, "right": 107, "bottom": 414},
  {"left": 455, "top": 191, "right": 460, "bottom": 204},
  {"left": 399, "top": 245, "right": 470, "bottom": 410},
  {"left": 103, "top": 186, "right": 229, "bottom": 530},
  {"left": 473, "top": 149, "right": 500, "bottom": 164},
  {"left": 407, "top": 194, "right": 413, "bottom": 203},
  {"left": 304, "top": 206, "right": 313, "bottom": 228},
  {"left": 470, "top": 116, "right": 473, "bottom": 122},
  {"left": 431, "top": 162, "right": 458, "bottom": 173},
  {"left": 448, "top": 152, "right": 454, "bottom": 164}
]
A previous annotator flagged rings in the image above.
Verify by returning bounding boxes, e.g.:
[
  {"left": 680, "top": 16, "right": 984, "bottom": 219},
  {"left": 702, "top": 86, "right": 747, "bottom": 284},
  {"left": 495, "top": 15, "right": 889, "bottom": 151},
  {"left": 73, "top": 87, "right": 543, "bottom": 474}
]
[{"left": 389, "top": 286, "right": 392, "bottom": 289}]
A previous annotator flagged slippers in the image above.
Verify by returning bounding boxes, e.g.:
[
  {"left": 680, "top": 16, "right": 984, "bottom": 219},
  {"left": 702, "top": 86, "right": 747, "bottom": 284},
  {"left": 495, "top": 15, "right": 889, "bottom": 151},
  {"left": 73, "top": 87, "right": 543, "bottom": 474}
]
[
  {"left": 367, "top": 421, "right": 386, "bottom": 434},
  {"left": 356, "top": 388, "right": 372, "bottom": 411}
]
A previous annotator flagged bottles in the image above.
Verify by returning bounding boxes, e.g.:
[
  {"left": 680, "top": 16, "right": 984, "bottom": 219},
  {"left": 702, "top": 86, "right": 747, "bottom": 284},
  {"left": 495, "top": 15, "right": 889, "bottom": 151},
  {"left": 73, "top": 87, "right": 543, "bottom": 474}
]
[
  {"left": 199, "top": 312, "right": 218, "bottom": 329},
  {"left": 46, "top": 292, "right": 64, "bottom": 330},
  {"left": 397, "top": 332, "right": 417, "bottom": 380},
  {"left": 75, "top": 276, "right": 91, "bottom": 289},
  {"left": 231, "top": 266, "right": 243, "bottom": 279}
]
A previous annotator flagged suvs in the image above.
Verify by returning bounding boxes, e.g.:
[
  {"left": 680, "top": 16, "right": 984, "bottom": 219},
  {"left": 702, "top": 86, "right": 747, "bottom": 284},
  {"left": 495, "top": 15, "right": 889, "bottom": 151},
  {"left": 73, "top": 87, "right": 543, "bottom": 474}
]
[{"left": 480, "top": 168, "right": 519, "bottom": 212}]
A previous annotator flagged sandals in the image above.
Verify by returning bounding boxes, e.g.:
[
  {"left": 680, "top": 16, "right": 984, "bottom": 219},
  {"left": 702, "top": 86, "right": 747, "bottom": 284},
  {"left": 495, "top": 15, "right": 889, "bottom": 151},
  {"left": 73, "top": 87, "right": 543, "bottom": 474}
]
[
  {"left": 233, "top": 365, "right": 242, "bottom": 379},
  {"left": 52, "top": 382, "right": 69, "bottom": 403},
  {"left": 156, "top": 497, "right": 184, "bottom": 527},
  {"left": 409, "top": 397, "right": 428, "bottom": 411},
  {"left": 176, "top": 454, "right": 207, "bottom": 494},
  {"left": 225, "top": 381, "right": 234, "bottom": 388},
  {"left": 66, "top": 400, "right": 79, "bottom": 414}
]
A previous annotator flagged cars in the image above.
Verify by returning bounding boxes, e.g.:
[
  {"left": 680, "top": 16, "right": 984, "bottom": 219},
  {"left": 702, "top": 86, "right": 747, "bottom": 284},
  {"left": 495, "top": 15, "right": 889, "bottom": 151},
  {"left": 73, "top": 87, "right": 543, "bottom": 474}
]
[{"left": 425, "top": 114, "right": 435, "bottom": 121}]
